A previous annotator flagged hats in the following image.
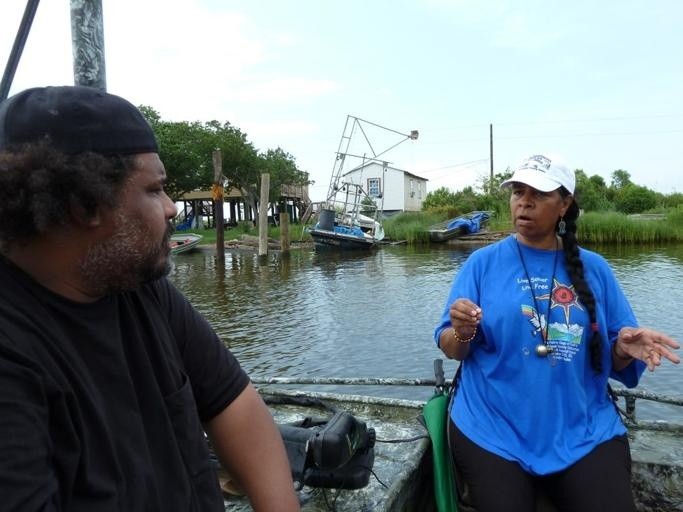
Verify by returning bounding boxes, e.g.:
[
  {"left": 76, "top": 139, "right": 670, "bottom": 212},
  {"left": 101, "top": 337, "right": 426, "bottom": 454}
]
[
  {"left": 0, "top": 84, "right": 159, "bottom": 159},
  {"left": 499, "top": 153, "right": 576, "bottom": 197}
]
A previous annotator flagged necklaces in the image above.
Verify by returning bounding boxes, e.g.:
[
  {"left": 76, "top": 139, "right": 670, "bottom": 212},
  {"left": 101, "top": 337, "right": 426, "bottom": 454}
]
[{"left": 513, "top": 232, "right": 560, "bottom": 357}]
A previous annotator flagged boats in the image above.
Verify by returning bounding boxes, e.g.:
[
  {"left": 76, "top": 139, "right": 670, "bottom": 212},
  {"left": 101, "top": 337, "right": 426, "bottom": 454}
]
[
  {"left": 421, "top": 208, "right": 496, "bottom": 242},
  {"left": 311, "top": 245, "right": 380, "bottom": 281},
  {"left": 306, "top": 113, "right": 419, "bottom": 250},
  {"left": 168, "top": 233, "right": 203, "bottom": 255}
]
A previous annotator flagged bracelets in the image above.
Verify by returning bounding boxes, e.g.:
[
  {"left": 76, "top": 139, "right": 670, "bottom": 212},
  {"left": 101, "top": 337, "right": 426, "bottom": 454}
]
[
  {"left": 451, "top": 325, "right": 477, "bottom": 344},
  {"left": 612, "top": 343, "right": 634, "bottom": 361}
]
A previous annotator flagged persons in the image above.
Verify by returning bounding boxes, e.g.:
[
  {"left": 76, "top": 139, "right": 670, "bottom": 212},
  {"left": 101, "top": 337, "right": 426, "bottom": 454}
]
[
  {"left": 432, "top": 149, "right": 683, "bottom": 511},
  {"left": 0, "top": 84, "right": 305, "bottom": 510}
]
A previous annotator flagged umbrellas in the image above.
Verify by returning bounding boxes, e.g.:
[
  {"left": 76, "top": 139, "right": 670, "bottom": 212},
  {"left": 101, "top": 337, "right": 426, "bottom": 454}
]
[{"left": 417, "top": 355, "right": 460, "bottom": 511}]
